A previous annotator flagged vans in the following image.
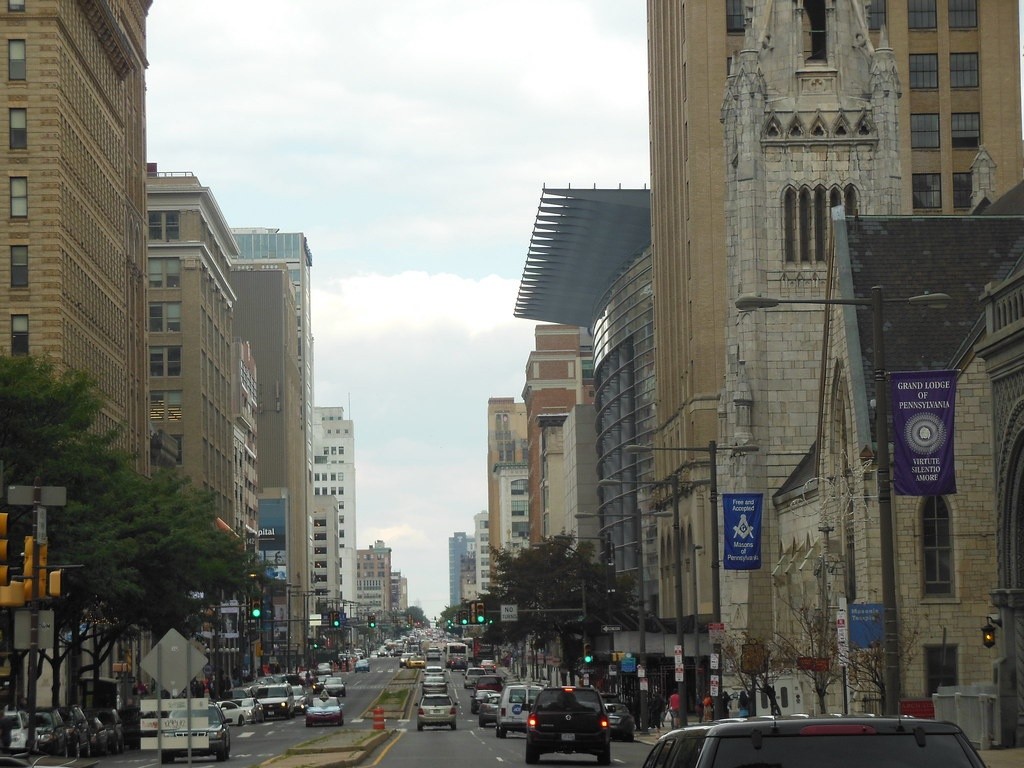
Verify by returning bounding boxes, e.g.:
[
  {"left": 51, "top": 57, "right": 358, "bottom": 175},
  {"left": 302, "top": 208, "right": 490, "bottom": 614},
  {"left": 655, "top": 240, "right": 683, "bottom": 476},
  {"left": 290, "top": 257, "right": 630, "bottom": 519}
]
[
  {"left": 638, "top": 715, "right": 987, "bottom": 768},
  {"left": 495, "top": 686, "right": 544, "bottom": 738}
]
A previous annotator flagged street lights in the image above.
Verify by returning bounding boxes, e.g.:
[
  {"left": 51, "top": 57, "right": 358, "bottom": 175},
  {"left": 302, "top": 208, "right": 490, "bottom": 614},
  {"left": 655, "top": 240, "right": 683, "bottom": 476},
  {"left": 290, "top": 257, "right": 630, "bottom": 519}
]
[
  {"left": 574, "top": 509, "right": 675, "bottom": 736},
  {"left": 622, "top": 439, "right": 761, "bottom": 724},
  {"left": 596, "top": 476, "right": 716, "bottom": 728},
  {"left": 556, "top": 532, "right": 615, "bottom": 652},
  {"left": 534, "top": 541, "right": 590, "bottom": 676}
]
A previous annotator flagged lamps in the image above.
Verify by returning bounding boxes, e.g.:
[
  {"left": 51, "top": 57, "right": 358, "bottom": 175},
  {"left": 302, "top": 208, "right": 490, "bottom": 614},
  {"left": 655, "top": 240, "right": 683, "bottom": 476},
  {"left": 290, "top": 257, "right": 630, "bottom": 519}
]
[
  {"left": 870, "top": 397, "right": 876, "bottom": 412},
  {"left": 694, "top": 544, "right": 703, "bottom": 556},
  {"left": 980, "top": 615, "right": 1003, "bottom": 649}
]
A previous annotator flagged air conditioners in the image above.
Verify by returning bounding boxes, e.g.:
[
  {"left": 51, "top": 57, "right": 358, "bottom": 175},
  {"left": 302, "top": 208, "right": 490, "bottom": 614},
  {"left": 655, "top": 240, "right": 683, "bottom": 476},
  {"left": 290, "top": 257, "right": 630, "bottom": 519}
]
[{"left": 183, "top": 258, "right": 196, "bottom": 270}]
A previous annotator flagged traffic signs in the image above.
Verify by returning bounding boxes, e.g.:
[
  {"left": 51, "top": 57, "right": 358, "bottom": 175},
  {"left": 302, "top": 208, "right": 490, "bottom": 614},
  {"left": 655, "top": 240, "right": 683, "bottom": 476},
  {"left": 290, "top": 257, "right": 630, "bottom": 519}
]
[{"left": 601, "top": 625, "right": 619, "bottom": 633}]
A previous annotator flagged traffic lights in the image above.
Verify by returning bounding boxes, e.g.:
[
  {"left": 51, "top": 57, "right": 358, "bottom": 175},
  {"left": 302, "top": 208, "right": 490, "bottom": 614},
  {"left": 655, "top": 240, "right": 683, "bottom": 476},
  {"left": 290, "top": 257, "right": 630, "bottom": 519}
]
[
  {"left": 50, "top": 568, "right": 67, "bottom": 598},
  {"left": 369, "top": 614, "right": 375, "bottom": 628},
  {"left": 461, "top": 610, "right": 468, "bottom": 625},
  {"left": 476, "top": 602, "right": 486, "bottom": 624},
  {"left": 0, "top": 511, "right": 13, "bottom": 587},
  {"left": 448, "top": 618, "right": 454, "bottom": 629},
  {"left": 335, "top": 614, "right": 340, "bottom": 628},
  {"left": 253, "top": 596, "right": 261, "bottom": 618},
  {"left": 582, "top": 641, "right": 592, "bottom": 663}
]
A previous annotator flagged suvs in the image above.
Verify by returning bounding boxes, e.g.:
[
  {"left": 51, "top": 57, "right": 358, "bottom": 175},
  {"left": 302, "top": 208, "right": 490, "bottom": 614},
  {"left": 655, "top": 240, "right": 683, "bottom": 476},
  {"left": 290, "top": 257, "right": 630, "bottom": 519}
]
[{"left": 521, "top": 685, "right": 613, "bottom": 766}]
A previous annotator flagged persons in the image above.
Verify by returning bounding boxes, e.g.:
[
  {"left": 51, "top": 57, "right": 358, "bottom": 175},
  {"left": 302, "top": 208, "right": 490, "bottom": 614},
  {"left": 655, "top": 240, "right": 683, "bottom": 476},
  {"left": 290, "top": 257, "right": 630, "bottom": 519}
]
[
  {"left": 625, "top": 690, "right": 751, "bottom": 732},
  {"left": 190, "top": 663, "right": 288, "bottom": 698}
]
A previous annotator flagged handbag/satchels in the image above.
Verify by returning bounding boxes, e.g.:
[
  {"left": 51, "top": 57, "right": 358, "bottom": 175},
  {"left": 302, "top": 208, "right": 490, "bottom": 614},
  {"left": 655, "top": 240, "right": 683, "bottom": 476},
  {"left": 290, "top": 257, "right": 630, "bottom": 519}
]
[
  {"left": 739, "top": 709, "right": 749, "bottom": 717},
  {"left": 665, "top": 711, "right": 672, "bottom": 722}
]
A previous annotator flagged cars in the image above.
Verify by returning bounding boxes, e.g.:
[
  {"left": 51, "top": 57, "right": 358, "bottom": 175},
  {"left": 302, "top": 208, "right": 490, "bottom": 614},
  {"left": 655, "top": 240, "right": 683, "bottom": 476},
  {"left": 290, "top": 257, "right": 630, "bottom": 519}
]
[
  {"left": 605, "top": 703, "right": 637, "bottom": 743},
  {"left": 1, "top": 703, "right": 157, "bottom": 761},
  {"left": 161, "top": 701, "right": 234, "bottom": 759},
  {"left": 216, "top": 632, "right": 407, "bottom": 729},
  {"left": 400, "top": 627, "right": 506, "bottom": 731}
]
